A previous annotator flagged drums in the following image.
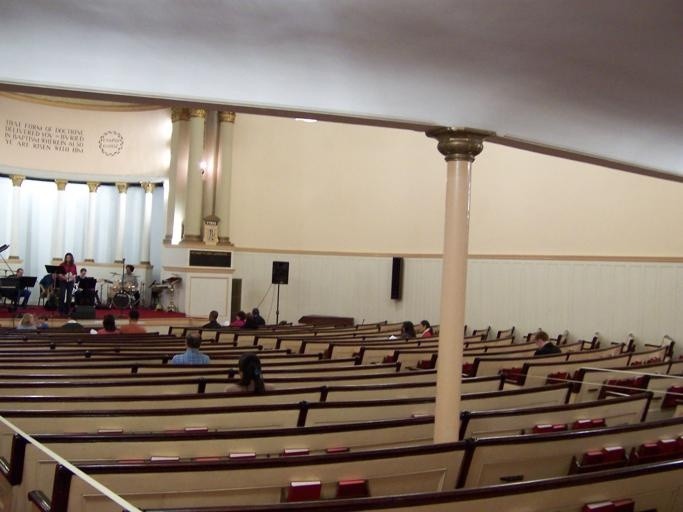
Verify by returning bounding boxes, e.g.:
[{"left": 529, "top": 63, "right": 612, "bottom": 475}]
[
  {"left": 112, "top": 292, "right": 131, "bottom": 309},
  {"left": 112, "top": 280, "right": 122, "bottom": 292},
  {"left": 124, "top": 280, "right": 135, "bottom": 291}
]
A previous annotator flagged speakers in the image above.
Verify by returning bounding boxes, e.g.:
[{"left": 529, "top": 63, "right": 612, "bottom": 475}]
[
  {"left": 271, "top": 261, "right": 289, "bottom": 284},
  {"left": 390, "top": 257, "right": 403, "bottom": 299},
  {"left": 71, "top": 306, "right": 96, "bottom": 319}
]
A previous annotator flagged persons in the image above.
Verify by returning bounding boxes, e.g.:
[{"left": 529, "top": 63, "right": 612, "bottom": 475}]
[
  {"left": 201, "top": 311, "right": 221, "bottom": 329},
  {"left": 73, "top": 268, "right": 88, "bottom": 291},
  {"left": 121, "top": 265, "right": 140, "bottom": 307},
  {"left": 35, "top": 314, "right": 49, "bottom": 328},
  {"left": 241, "top": 313, "right": 258, "bottom": 329},
  {"left": 16, "top": 313, "right": 37, "bottom": 330},
  {"left": 96, "top": 315, "right": 118, "bottom": 335},
  {"left": 8, "top": 268, "right": 31, "bottom": 308},
  {"left": 38, "top": 271, "right": 57, "bottom": 309},
  {"left": 60, "top": 311, "right": 83, "bottom": 328},
  {"left": 119, "top": 310, "right": 147, "bottom": 334},
  {"left": 397, "top": 320, "right": 416, "bottom": 339},
  {"left": 533, "top": 330, "right": 562, "bottom": 355},
  {"left": 223, "top": 353, "right": 274, "bottom": 392},
  {"left": 252, "top": 308, "right": 265, "bottom": 324},
  {"left": 229, "top": 311, "right": 246, "bottom": 327},
  {"left": 417, "top": 319, "right": 433, "bottom": 338},
  {"left": 171, "top": 332, "right": 210, "bottom": 364},
  {"left": 57, "top": 252, "right": 76, "bottom": 315}
]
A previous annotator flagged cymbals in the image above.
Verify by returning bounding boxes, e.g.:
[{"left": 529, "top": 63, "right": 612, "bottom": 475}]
[
  {"left": 109, "top": 271, "right": 120, "bottom": 276},
  {"left": 127, "top": 275, "right": 140, "bottom": 278}
]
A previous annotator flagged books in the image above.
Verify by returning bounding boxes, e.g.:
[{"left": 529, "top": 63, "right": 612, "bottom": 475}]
[
  {"left": 227, "top": 453, "right": 256, "bottom": 460},
  {"left": 572, "top": 419, "right": 590, "bottom": 430},
  {"left": 580, "top": 452, "right": 601, "bottom": 467},
  {"left": 96, "top": 429, "right": 123, "bottom": 435},
  {"left": 29, "top": 491, "right": 51, "bottom": 512},
  {"left": 165, "top": 428, "right": 183, "bottom": 434},
  {"left": 64, "top": 432, "right": 86, "bottom": 437},
  {"left": 0, "top": 463, "right": 9, "bottom": 474},
  {"left": 602, "top": 446, "right": 623, "bottom": 462},
  {"left": 184, "top": 427, "right": 207, "bottom": 432},
  {"left": 0, "top": 456, "right": 11, "bottom": 471},
  {"left": 552, "top": 425, "right": 566, "bottom": 431},
  {"left": 637, "top": 444, "right": 656, "bottom": 457},
  {"left": 325, "top": 447, "right": 349, "bottom": 454},
  {"left": 579, "top": 500, "right": 614, "bottom": 512},
  {"left": 656, "top": 440, "right": 675, "bottom": 456},
  {"left": 335, "top": 480, "right": 366, "bottom": 498},
  {"left": 117, "top": 460, "right": 144, "bottom": 464},
  {"left": 283, "top": 449, "right": 310, "bottom": 457},
  {"left": 411, "top": 414, "right": 424, "bottom": 418},
  {"left": 150, "top": 456, "right": 179, "bottom": 463},
  {"left": 675, "top": 435, "right": 682, "bottom": 454},
  {"left": 34, "top": 491, "right": 51, "bottom": 511},
  {"left": 611, "top": 498, "right": 634, "bottom": 512},
  {"left": 533, "top": 424, "right": 552, "bottom": 433},
  {"left": 285, "top": 479, "right": 321, "bottom": 502},
  {"left": 591, "top": 419, "right": 603, "bottom": 428},
  {"left": 191, "top": 457, "right": 220, "bottom": 464}
]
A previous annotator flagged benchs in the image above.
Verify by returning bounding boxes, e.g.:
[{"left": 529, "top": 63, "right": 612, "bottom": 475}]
[{"left": 0, "top": 317, "right": 682, "bottom": 512}]
